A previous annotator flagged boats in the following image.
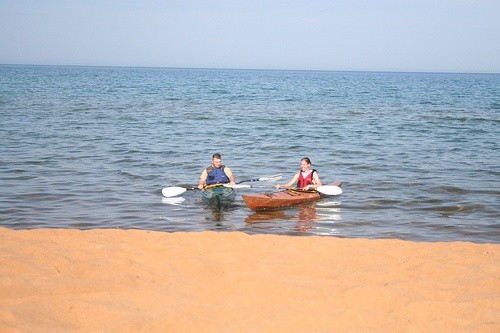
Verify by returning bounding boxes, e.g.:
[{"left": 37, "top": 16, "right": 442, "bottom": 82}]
[
  {"left": 202, "top": 184, "right": 236, "bottom": 214},
  {"left": 241, "top": 181, "right": 343, "bottom": 212}
]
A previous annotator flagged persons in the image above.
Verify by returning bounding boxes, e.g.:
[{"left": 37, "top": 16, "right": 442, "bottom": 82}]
[
  {"left": 274, "top": 156, "right": 323, "bottom": 191},
  {"left": 198, "top": 153, "right": 236, "bottom": 189}
]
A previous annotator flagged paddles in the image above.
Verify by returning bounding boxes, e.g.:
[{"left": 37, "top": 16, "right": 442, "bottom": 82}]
[
  {"left": 162, "top": 173, "right": 283, "bottom": 197},
  {"left": 222, "top": 184, "right": 343, "bottom": 195}
]
